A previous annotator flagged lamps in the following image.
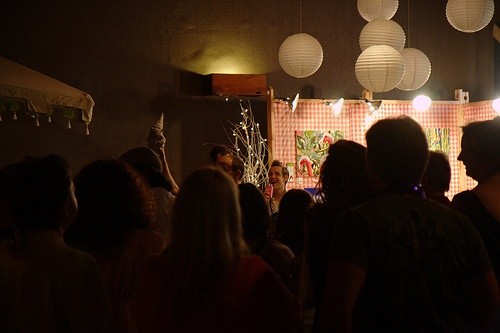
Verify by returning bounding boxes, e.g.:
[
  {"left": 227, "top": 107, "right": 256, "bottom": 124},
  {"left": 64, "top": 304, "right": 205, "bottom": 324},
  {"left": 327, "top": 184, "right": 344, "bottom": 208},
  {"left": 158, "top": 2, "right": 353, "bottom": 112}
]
[
  {"left": 277, "top": 0, "right": 324, "bottom": 79},
  {"left": 446, "top": 0, "right": 494, "bottom": 33},
  {"left": 357, "top": 0, "right": 431, "bottom": 94},
  {"left": 323, "top": 98, "right": 345, "bottom": 118},
  {"left": 365, "top": 100, "right": 382, "bottom": 116},
  {"left": 274, "top": 93, "right": 301, "bottom": 114}
]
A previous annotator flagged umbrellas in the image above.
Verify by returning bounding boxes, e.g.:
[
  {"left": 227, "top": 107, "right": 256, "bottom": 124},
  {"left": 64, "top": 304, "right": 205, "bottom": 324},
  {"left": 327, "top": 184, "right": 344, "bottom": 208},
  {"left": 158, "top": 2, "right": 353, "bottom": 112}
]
[{"left": 0, "top": 56, "right": 96, "bottom": 136}]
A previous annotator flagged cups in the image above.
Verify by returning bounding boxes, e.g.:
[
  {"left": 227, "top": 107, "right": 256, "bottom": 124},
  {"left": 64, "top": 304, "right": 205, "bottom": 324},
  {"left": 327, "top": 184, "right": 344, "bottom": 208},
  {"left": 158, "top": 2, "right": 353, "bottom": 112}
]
[
  {"left": 455, "top": 89, "right": 463, "bottom": 101},
  {"left": 266, "top": 184, "right": 273, "bottom": 199}
]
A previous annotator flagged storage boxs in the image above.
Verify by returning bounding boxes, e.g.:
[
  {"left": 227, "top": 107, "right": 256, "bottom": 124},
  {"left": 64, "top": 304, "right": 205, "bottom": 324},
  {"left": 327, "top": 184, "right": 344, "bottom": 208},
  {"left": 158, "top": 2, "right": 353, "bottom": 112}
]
[{"left": 207, "top": 73, "right": 267, "bottom": 96}]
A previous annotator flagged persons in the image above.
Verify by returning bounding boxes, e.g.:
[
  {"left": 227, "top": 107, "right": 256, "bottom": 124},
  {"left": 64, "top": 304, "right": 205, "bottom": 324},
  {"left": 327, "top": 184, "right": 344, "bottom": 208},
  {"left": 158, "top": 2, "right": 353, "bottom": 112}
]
[
  {"left": 319, "top": 115, "right": 500, "bottom": 333},
  {"left": 417, "top": 152, "right": 451, "bottom": 207},
  {"left": 450, "top": 120, "right": 500, "bottom": 287},
  {"left": 0, "top": 136, "right": 315, "bottom": 333},
  {"left": 305, "top": 141, "right": 377, "bottom": 333}
]
